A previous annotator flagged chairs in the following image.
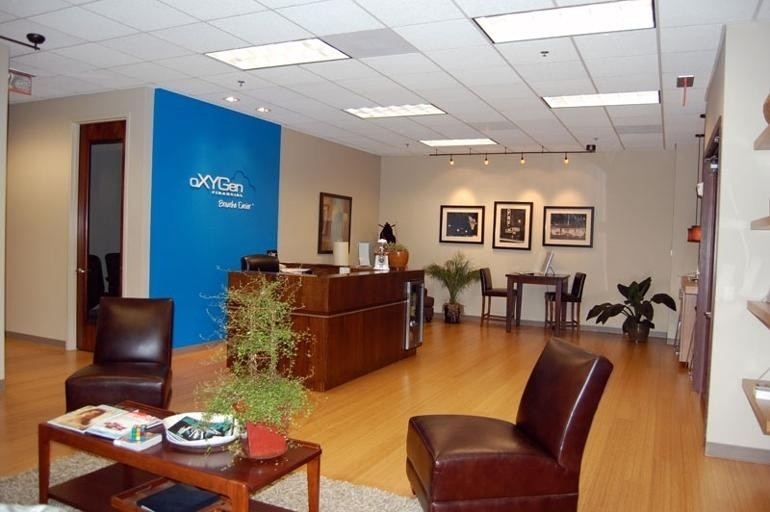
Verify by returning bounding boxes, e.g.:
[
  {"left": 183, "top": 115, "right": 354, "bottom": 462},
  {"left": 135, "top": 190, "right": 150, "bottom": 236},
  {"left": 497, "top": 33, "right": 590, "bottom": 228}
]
[
  {"left": 406, "top": 336, "right": 615, "bottom": 512},
  {"left": 66, "top": 296, "right": 175, "bottom": 413},
  {"left": 480, "top": 267, "right": 517, "bottom": 328},
  {"left": 545, "top": 272, "right": 587, "bottom": 333},
  {"left": 240, "top": 255, "right": 280, "bottom": 273}
]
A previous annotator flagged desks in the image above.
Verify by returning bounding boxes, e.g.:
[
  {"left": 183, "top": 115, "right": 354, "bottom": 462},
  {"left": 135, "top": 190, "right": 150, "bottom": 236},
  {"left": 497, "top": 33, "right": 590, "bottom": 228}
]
[
  {"left": 227, "top": 263, "right": 425, "bottom": 392},
  {"left": 506, "top": 273, "right": 570, "bottom": 332},
  {"left": 38, "top": 401, "right": 321, "bottom": 512}
]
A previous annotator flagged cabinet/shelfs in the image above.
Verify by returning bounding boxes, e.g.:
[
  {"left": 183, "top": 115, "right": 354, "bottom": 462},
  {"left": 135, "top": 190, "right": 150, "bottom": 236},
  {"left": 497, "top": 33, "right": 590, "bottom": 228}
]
[
  {"left": 741, "top": 101, "right": 770, "bottom": 438},
  {"left": 676, "top": 276, "right": 700, "bottom": 366}
]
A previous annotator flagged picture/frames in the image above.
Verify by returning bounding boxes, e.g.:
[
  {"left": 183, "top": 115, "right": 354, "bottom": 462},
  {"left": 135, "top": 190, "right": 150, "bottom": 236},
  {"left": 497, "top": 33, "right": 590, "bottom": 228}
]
[
  {"left": 440, "top": 204, "right": 485, "bottom": 244},
  {"left": 493, "top": 201, "right": 533, "bottom": 250},
  {"left": 318, "top": 192, "right": 353, "bottom": 253},
  {"left": 543, "top": 206, "right": 594, "bottom": 247}
]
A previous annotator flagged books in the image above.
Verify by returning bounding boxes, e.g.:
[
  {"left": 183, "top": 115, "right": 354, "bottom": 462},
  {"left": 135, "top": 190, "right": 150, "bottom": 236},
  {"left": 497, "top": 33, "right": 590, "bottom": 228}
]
[
  {"left": 137, "top": 483, "right": 219, "bottom": 512},
  {"left": 47, "top": 405, "right": 123, "bottom": 434},
  {"left": 89, "top": 410, "right": 162, "bottom": 440}
]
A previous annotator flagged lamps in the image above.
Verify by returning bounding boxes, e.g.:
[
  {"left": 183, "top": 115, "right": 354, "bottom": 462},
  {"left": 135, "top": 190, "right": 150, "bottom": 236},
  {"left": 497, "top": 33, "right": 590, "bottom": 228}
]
[
  {"left": 563, "top": 158, "right": 568, "bottom": 164},
  {"left": 520, "top": 157, "right": 525, "bottom": 165},
  {"left": 449, "top": 159, "right": 454, "bottom": 165},
  {"left": 484, "top": 159, "right": 488, "bottom": 165}
]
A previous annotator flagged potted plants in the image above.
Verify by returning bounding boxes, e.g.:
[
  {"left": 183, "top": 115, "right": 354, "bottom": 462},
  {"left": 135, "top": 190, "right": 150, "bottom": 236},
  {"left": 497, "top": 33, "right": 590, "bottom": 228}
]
[
  {"left": 195, "top": 270, "right": 315, "bottom": 460},
  {"left": 385, "top": 242, "right": 409, "bottom": 269},
  {"left": 587, "top": 277, "right": 676, "bottom": 343},
  {"left": 424, "top": 255, "right": 478, "bottom": 325}
]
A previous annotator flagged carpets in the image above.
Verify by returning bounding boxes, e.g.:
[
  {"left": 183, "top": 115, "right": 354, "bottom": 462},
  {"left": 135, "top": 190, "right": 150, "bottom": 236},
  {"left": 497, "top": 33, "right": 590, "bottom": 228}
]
[{"left": 0, "top": 452, "right": 425, "bottom": 511}]
